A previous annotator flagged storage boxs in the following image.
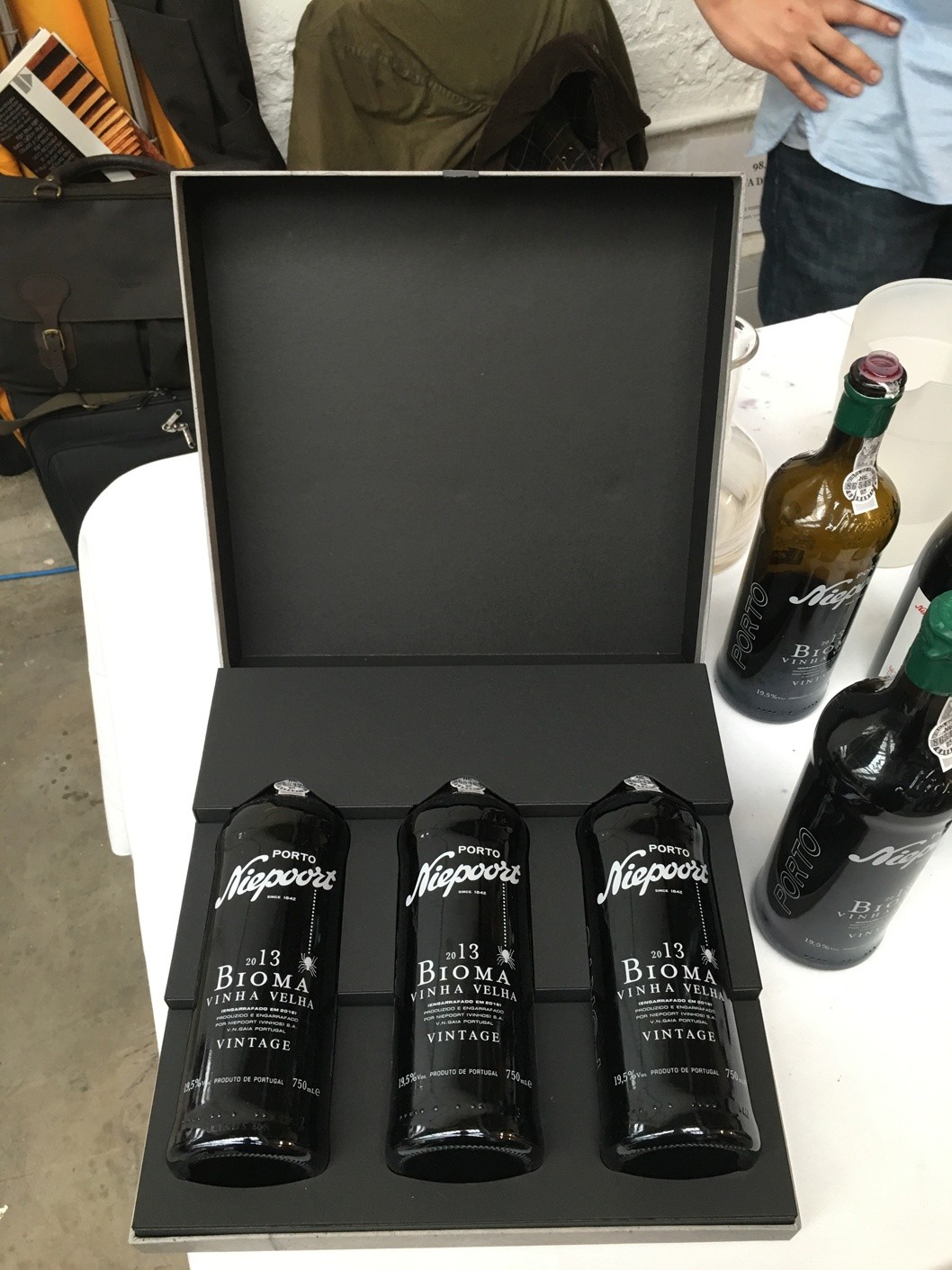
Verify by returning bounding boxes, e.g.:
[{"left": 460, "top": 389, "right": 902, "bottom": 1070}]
[{"left": 126, "top": 167, "right": 805, "bottom": 1241}]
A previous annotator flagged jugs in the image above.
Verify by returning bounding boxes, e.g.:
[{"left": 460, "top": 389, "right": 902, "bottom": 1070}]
[{"left": 829, "top": 278, "right": 952, "bottom": 570}]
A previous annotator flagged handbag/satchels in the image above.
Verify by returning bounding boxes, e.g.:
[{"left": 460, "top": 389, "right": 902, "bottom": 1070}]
[{"left": 0, "top": 155, "right": 263, "bottom": 566}]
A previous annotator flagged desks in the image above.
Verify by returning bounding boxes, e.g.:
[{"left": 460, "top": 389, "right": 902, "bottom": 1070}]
[{"left": 78, "top": 285, "right": 948, "bottom": 1270}]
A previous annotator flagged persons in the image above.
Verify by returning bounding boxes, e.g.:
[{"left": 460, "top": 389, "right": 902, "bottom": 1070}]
[{"left": 692, "top": 0, "right": 952, "bottom": 326}]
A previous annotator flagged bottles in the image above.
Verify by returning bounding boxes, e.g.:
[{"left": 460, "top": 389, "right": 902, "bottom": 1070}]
[
  {"left": 385, "top": 782, "right": 543, "bottom": 1184},
  {"left": 865, "top": 512, "right": 952, "bottom": 680},
  {"left": 166, "top": 781, "right": 349, "bottom": 1188},
  {"left": 711, "top": 313, "right": 769, "bottom": 577},
  {"left": 577, "top": 778, "right": 761, "bottom": 1180},
  {"left": 715, "top": 349, "right": 908, "bottom": 727},
  {"left": 750, "top": 591, "right": 951, "bottom": 972}
]
